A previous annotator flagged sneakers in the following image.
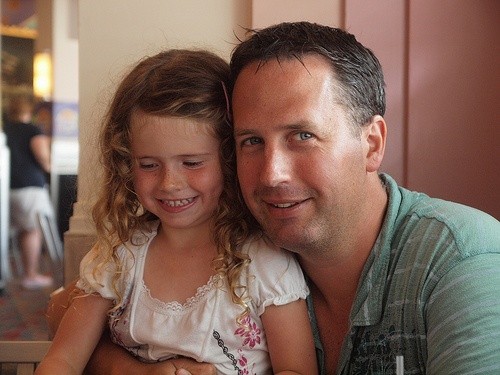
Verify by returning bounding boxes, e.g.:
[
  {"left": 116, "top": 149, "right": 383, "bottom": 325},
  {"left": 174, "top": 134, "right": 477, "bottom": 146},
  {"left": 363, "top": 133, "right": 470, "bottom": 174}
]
[{"left": 22, "top": 273, "right": 52, "bottom": 289}]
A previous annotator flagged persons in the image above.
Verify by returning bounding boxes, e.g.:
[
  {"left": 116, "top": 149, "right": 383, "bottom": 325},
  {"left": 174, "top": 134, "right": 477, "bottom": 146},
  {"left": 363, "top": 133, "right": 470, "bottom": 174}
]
[
  {"left": 3, "top": 92, "right": 53, "bottom": 290},
  {"left": 33, "top": 44, "right": 319, "bottom": 375},
  {"left": 47, "top": 21, "right": 500, "bottom": 375}
]
